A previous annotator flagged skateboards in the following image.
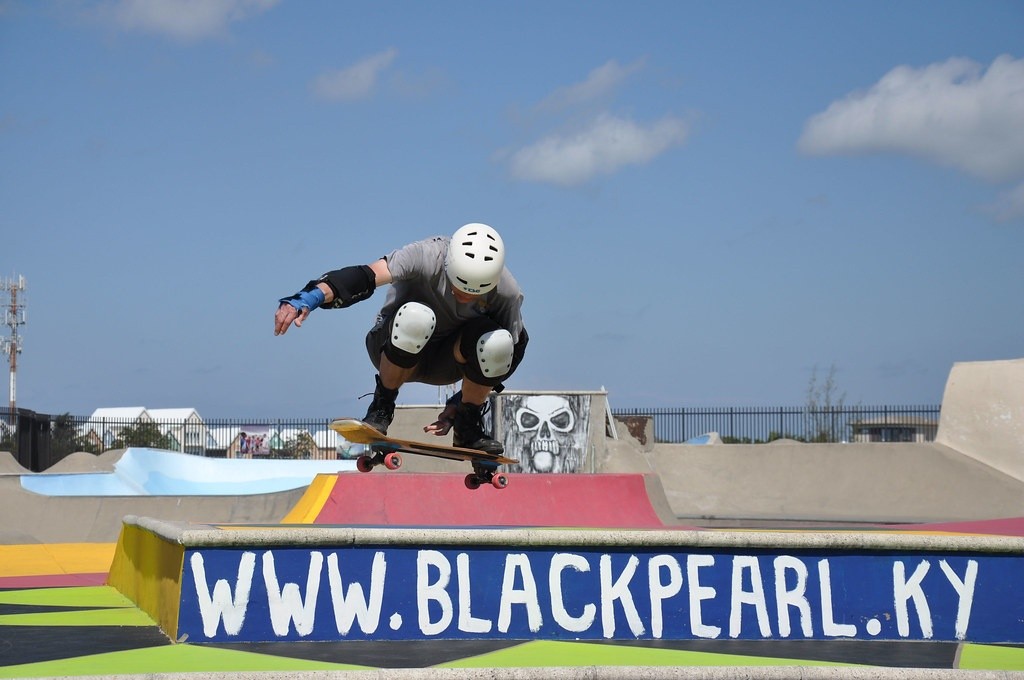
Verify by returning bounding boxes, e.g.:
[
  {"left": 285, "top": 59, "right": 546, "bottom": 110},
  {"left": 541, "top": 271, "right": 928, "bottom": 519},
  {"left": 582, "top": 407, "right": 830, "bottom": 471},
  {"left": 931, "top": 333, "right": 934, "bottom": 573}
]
[{"left": 329, "top": 420, "right": 520, "bottom": 490}]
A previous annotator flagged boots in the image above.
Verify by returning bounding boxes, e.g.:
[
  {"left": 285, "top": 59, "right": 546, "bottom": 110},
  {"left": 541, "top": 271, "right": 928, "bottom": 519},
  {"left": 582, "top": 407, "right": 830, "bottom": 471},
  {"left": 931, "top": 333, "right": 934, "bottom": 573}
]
[
  {"left": 452, "top": 398, "right": 505, "bottom": 453},
  {"left": 356, "top": 374, "right": 399, "bottom": 436}
]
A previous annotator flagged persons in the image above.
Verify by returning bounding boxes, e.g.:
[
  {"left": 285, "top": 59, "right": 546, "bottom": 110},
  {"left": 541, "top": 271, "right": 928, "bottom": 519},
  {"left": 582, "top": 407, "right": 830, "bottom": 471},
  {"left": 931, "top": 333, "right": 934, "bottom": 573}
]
[
  {"left": 274, "top": 222, "right": 529, "bottom": 453},
  {"left": 247, "top": 434, "right": 269, "bottom": 452},
  {"left": 240, "top": 432, "right": 249, "bottom": 458}
]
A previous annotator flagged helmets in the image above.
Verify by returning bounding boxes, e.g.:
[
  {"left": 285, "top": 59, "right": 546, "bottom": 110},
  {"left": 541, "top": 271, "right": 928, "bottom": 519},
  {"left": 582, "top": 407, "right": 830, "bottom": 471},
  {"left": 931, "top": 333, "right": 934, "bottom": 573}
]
[{"left": 445, "top": 223, "right": 504, "bottom": 295}]
